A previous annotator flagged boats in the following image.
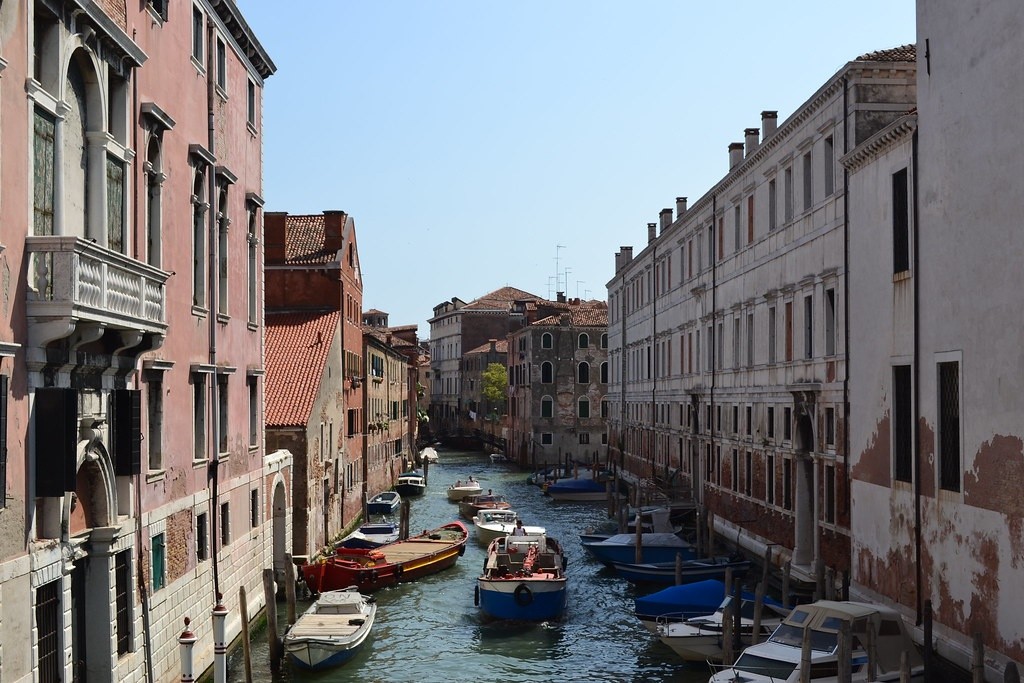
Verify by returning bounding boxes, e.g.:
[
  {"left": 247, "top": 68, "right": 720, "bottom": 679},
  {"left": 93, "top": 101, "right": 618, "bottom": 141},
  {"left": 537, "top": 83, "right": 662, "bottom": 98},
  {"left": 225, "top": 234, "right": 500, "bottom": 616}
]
[
  {"left": 580, "top": 532, "right": 692, "bottom": 577},
  {"left": 472, "top": 509, "right": 546, "bottom": 547},
  {"left": 367, "top": 492, "right": 401, "bottom": 515},
  {"left": 333, "top": 521, "right": 400, "bottom": 549},
  {"left": 394, "top": 472, "right": 426, "bottom": 497},
  {"left": 530, "top": 462, "right": 590, "bottom": 482},
  {"left": 283, "top": 585, "right": 377, "bottom": 674},
  {"left": 419, "top": 447, "right": 438, "bottom": 464},
  {"left": 545, "top": 478, "right": 627, "bottom": 501},
  {"left": 447, "top": 482, "right": 484, "bottom": 503},
  {"left": 705, "top": 600, "right": 924, "bottom": 683},
  {"left": 634, "top": 579, "right": 794, "bottom": 640},
  {"left": 458, "top": 493, "right": 511, "bottom": 522},
  {"left": 578, "top": 502, "right": 698, "bottom": 559},
  {"left": 475, "top": 534, "right": 568, "bottom": 621},
  {"left": 656, "top": 593, "right": 792, "bottom": 662},
  {"left": 301, "top": 521, "right": 469, "bottom": 594},
  {"left": 490, "top": 454, "right": 507, "bottom": 464},
  {"left": 612, "top": 558, "right": 751, "bottom": 594}
]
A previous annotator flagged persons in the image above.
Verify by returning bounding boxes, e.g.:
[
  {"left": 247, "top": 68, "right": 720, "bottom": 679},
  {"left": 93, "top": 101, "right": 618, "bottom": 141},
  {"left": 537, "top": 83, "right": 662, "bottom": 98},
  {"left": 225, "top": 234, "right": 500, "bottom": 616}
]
[
  {"left": 455, "top": 480, "right": 461, "bottom": 487},
  {"left": 511, "top": 520, "right": 528, "bottom": 536},
  {"left": 488, "top": 489, "right": 492, "bottom": 495}
]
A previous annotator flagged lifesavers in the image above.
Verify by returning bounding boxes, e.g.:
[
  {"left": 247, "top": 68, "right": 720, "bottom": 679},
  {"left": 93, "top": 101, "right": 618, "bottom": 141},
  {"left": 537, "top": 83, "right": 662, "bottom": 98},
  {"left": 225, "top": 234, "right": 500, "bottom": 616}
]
[{"left": 514, "top": 584, "right": 534, "bottom": 606}]
